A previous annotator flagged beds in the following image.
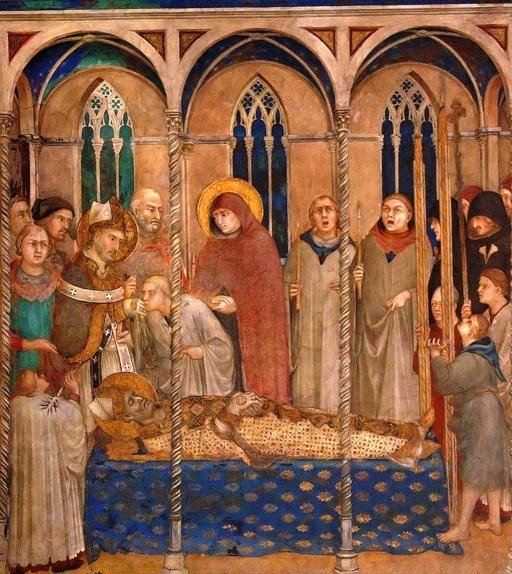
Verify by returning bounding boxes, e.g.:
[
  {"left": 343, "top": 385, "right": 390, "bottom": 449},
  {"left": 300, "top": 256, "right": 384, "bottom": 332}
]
[{"left": 83, "top": 430, "right": 464, "bottom": 565}]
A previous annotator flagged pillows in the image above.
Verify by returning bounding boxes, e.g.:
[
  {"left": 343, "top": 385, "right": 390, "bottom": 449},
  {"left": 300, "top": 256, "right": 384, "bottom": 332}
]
[{"left": 93, "top": 424, "right": 144, "bottom": 461}]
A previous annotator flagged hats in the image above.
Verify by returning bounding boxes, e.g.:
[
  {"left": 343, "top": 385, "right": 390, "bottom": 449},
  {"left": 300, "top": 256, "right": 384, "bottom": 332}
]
[
  {"left": 91, "top": 370, "right": 159, "bottom": 439},
  {"left": 76, "top": 203, "right": 139, "bottom": 264},
  {"left": 196, "top": 175, "right": 265, "bottom": 237}
]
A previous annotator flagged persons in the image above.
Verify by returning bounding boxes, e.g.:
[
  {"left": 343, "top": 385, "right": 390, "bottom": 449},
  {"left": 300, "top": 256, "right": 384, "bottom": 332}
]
[
  {"left": 135, "top": 273, "right": 242, "bottom": 394},
  {"left": 351, "top": 193, "right": 431, "bottom": 432},
  {"left": 25, "top": 192, "right": 75, "bottom": 267},
  {"left": 5, "top": 220, "right": 59, "bottom": 380},
  {"left": 8, "top": 194, "right": 33, "bottom": 235},
  {"left": 184, "top": 178, "right": 293, "bottom": 410},
  {"left": 89, "top": 371, "right": 442, "bottom": 470},
  {"left": 112, "top": 186, "right": 187, "bottom": 300},
  {"left": 4, "top": 365, "right": 89, "bottom": 573},
  {"left": 412, "top": 171, "right": 512, "bottom": 543},
  {"left": 43, "top": 197, "right": 139, "bottom": 399},
  {"left": 285, "top": 194, "right": 359, "bottom": 412}
]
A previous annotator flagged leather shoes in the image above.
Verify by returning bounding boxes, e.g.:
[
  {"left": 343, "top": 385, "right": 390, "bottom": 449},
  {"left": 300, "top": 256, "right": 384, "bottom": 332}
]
[{"left": 51, "top": 559, "right": 83, "bottom": 573}]
